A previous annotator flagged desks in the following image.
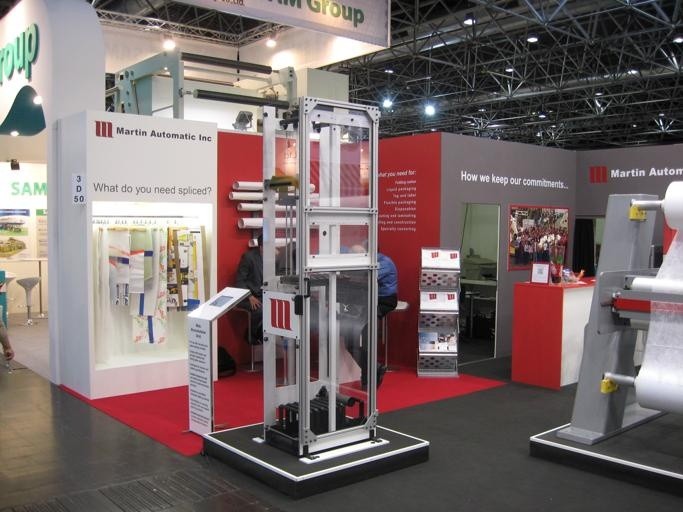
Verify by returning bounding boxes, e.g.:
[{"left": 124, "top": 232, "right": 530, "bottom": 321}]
[{"left": 459, "top": 277, "right": 496, "bottom": 342}]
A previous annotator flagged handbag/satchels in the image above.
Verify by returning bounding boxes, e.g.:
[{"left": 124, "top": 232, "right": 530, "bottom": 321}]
[{"left": 218, "top": 347, "right": 236, "bottom": 376}]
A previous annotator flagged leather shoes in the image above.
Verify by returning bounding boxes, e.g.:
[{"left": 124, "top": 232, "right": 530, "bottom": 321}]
[{"left": 360, "top": 361, "right": 386, "bottom": 390}]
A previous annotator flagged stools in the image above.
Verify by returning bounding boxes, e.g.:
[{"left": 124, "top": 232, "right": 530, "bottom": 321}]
[
  {"left": 230, "top": 305, "right": 261, "bottom": 374},
  {"left": 375, "top": 298, "right": 410, "bottom": 373}
]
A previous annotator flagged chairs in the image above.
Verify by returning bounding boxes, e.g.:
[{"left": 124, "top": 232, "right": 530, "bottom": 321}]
[{"left": 15, "top": 277, "right": 42, "bottom": 327}]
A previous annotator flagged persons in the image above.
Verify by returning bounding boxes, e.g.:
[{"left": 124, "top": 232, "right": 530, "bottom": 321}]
[
  {"left": 510, "top": 206, "right": 568, "bottom": 269},
  {"left": 0, "top": 320, "right": 14, "bottom": 361},
  {"left": 233, "top": 232, "right": 270, "bottom": 346},
  {"left": 341, "top": 244, "right": 399, "bottom": 390}
]
